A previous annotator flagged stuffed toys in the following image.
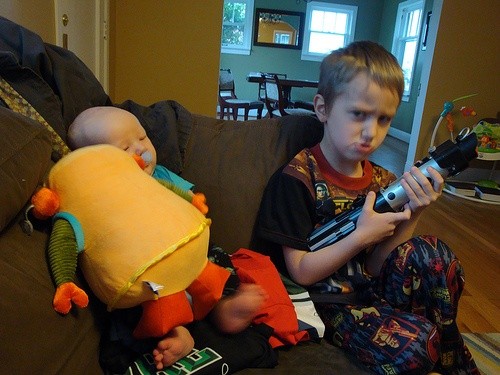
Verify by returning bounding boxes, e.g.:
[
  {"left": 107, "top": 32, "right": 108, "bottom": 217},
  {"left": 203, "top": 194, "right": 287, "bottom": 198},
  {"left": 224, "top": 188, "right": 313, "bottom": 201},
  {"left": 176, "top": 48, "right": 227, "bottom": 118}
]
[{"left": 24, "top": 142, "right": 231, "bottom": 340}]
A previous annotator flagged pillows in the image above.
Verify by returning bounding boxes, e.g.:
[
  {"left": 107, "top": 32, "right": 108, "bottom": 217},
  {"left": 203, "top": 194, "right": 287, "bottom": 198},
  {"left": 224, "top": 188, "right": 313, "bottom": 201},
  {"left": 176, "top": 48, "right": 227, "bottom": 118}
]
[{"left": 0, "top": 106, "right": 53, "bottom": 234}]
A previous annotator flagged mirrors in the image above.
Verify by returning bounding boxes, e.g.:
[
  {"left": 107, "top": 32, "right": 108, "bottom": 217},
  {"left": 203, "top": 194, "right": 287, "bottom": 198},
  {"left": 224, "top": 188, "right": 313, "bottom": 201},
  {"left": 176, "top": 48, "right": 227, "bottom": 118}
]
[{"left": 253, "top": 8, "right": 306, "bottom": 50}]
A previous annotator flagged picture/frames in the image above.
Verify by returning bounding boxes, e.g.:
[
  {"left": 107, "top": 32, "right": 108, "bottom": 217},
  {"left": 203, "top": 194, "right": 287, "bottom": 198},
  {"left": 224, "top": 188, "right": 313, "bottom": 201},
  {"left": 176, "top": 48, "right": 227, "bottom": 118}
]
[{"left": 421, "top": 11, "right": 432, "bottom": 51}]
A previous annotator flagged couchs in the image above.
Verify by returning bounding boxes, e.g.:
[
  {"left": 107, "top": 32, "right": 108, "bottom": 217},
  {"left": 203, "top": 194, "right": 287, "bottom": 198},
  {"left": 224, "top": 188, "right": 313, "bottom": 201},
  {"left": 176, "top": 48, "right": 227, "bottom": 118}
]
[{"left": 0, "top": 17, "right": 374, "bottom": 375}]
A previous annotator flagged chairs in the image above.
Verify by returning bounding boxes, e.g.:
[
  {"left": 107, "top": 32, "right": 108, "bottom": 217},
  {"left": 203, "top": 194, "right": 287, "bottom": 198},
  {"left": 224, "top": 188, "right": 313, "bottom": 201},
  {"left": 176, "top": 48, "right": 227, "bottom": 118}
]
[
  {"left": 261, "top": 73, "right": 317, "bottom": 118},
  {"left": 257, "top": 73, "right": 291, "bottom": 104},
  {"left": 218, "top": 69, "right": 264, "bottom": 121}
]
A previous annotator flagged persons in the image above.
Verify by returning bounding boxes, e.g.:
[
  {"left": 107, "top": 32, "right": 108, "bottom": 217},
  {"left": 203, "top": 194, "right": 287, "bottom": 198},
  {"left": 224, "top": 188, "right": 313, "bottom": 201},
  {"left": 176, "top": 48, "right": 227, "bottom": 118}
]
[
  {"left": 255, "top": 40, "right": 484, "bottom": 375},
  {"left": 68, "top": 105, "right": 266, "bottom": 371}
]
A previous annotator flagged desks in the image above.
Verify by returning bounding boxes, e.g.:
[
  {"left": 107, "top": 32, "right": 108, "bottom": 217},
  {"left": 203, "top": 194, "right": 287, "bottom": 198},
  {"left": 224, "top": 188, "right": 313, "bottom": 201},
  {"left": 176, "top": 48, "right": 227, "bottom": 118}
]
[{"left": 246, "top": 71, "right": 319, "bottom": 108}]
